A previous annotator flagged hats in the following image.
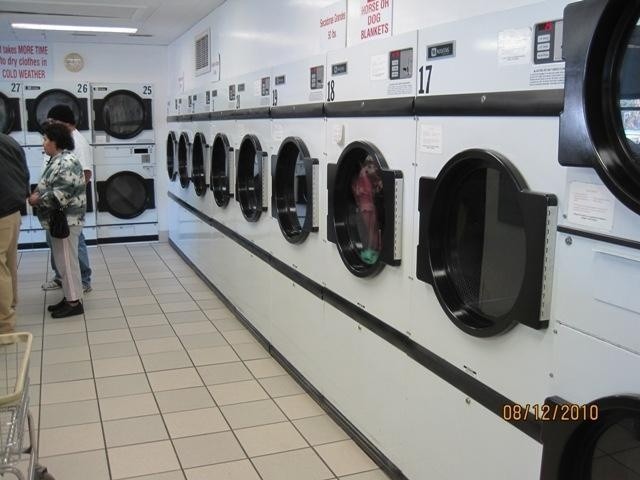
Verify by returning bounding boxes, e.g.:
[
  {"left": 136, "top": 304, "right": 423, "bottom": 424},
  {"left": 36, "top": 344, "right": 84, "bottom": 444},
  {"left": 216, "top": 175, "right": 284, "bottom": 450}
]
[{"left": 47, "top": 105, "right": 75, "bottom": 124}]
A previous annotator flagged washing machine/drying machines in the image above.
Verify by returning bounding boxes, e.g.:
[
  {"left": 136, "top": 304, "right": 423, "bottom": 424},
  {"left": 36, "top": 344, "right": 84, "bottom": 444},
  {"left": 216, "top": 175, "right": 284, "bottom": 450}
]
[
  {"left": 166, "top": 0, "right": 640, "bottom": 479},
  {"left": 1, "top": 78, "right": 158, "bottom": 241}
]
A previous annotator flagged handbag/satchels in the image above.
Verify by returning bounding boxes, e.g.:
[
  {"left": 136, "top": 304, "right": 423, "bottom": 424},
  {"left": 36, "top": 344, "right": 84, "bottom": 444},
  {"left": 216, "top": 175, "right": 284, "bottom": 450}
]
[{"left": 49, "top": 211, "right": 69, "bottom": 239}]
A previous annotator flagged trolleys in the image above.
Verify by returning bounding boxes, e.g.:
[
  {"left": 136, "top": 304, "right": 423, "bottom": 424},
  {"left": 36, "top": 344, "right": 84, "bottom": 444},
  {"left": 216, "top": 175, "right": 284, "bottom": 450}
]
[{"left": 0, "top": 333, "right": 53, "bottom": 480}]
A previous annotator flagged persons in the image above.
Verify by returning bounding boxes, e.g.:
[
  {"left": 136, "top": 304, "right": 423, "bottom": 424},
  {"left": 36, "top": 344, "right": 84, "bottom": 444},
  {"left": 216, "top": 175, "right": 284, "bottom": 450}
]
[
  {"left": 0, "top": 131, "right": 33, "bottom": 325},
  {"left": 27, "top": 123, "right": 88, "bottom": 318},
  {"left": 41, "top": 104, "right": 91, "bottom": 295}
]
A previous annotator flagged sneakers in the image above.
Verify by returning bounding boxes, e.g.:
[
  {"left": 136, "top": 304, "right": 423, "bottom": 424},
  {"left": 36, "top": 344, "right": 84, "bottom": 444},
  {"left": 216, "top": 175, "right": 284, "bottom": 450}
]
[
  {"left": 82, "top": 285, "right": 93, "bottom": 295},
  {"left": 48, "top": 297, "right": 84, "bottom": 318},
  {"left": 41, "top": 279, "right": 62, "bottom": 291}
]
